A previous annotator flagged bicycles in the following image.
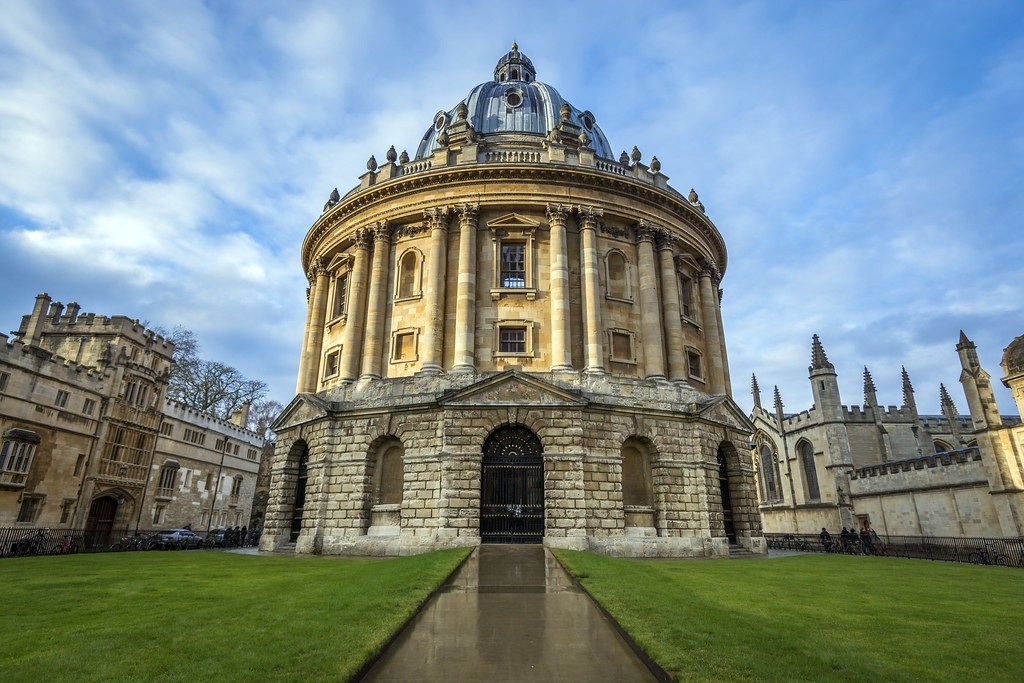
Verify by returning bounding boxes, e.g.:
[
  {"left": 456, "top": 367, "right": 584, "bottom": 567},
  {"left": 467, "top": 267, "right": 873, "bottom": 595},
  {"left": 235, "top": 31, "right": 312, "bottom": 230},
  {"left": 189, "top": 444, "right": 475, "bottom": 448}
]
[
  {"left": 14, "top": 525, "right": 258, "bottom": 557},
  {"left": 968, "top": 541, "right": 1015, "bottom": 567},
  {"left": 764, "top": 534, "right": 900, "bottom": 557}
]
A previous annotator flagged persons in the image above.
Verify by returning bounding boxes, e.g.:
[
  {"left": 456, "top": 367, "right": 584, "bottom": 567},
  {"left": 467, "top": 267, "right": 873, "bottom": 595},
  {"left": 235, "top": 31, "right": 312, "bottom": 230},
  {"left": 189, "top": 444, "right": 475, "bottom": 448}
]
[
  {"left": 183, "top": 523, "right": 192, "bottom": 532},
  {"left": 223, "top": 526, "right": 262, "bottom": 549},
  {"left": 820, "top": 527, "right": 882, "bottom": 556}
]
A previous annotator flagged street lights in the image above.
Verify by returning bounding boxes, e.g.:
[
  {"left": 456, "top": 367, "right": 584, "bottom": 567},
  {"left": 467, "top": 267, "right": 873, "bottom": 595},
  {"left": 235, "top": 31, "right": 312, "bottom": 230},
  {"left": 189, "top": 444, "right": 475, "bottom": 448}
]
[{"left": 205, "top": 434, "right": 232, "bottom": 539}]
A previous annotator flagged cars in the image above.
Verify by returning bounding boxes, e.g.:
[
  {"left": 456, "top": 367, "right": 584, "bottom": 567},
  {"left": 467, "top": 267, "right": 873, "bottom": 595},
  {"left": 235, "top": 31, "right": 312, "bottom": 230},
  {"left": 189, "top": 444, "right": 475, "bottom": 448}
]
[
  {"left": 206, "top": 527, "right": 228, "bottom": 547},
  {"left": 152, "top": 528, "right": 203, "bottom": 551}
]
